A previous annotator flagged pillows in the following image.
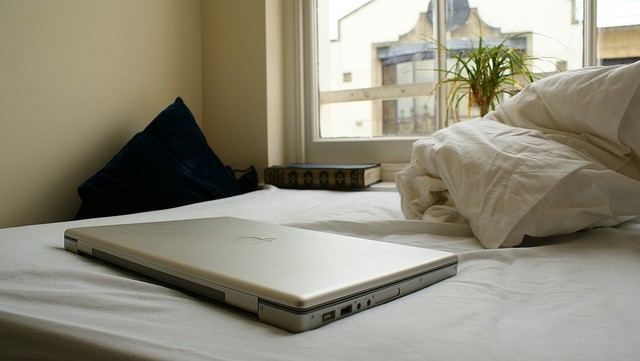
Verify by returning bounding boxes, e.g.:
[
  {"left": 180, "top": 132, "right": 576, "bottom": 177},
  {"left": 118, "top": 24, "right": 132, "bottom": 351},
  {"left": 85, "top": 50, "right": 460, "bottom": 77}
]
[{"left": 78, "top": 94, "right": 232, "bottom": 206}]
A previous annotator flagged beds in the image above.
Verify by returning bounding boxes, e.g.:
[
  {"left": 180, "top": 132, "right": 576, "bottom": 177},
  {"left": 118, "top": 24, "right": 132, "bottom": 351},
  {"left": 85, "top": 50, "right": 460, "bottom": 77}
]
[{"left": 1, "top": 185, "right": 640, "bottom": 361}]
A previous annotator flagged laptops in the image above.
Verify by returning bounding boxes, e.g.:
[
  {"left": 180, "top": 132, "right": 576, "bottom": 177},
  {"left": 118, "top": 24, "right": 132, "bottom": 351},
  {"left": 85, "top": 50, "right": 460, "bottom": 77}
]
[{"left": 63, "top": 216, "right": 459, "bottom": 334}]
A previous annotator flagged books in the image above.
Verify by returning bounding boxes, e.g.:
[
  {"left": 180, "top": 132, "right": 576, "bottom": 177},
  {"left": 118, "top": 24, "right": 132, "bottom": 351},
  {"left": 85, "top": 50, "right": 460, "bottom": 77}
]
[{"left": 263, "top": 164, "right": 383, "bottom": 188}]
